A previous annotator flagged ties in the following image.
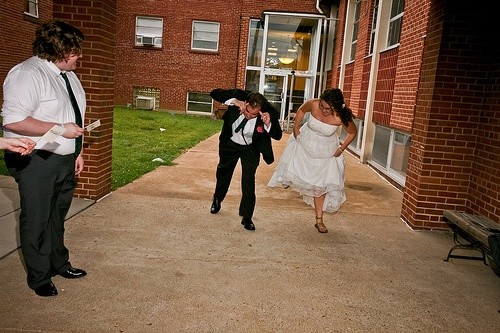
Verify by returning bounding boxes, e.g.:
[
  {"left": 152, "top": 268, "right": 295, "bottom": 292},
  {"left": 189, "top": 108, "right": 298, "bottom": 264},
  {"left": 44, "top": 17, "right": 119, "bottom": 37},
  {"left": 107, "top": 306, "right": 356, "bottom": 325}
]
[
  {"left": 235, "top": 118, "right": 248, "bottom": 133},
  {"left": 61, "top": 72, "right": 83, "bottom": 160}
]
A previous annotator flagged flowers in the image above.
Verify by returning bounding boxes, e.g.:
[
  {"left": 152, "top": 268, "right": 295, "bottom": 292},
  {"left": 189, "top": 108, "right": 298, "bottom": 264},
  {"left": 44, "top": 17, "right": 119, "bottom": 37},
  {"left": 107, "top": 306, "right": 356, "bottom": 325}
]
[{"left": 257, "top": 126, "right": 263, "bottom": 133}]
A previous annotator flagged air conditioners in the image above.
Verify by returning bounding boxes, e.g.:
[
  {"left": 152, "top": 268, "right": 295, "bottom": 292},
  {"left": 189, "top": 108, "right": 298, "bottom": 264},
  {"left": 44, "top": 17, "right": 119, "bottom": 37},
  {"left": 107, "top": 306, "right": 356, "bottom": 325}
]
[
  {"left": 142, "top": 37, "right": 155, "bottom": 45},
  {"left": 136, "top": 96, "right": 155, "bottom": 110}
]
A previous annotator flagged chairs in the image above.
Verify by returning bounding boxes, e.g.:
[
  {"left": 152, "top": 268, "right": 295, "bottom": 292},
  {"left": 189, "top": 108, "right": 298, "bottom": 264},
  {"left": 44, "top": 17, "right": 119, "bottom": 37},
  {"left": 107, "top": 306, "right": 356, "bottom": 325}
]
[{"left": 278, "top": 113, "right": 296, "bottom": 132}]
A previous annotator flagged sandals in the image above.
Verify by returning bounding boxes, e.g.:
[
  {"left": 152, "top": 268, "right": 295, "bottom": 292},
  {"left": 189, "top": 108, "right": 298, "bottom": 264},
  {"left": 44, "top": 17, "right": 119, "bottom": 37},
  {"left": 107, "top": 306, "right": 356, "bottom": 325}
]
[{"left": 314, "top": 216, "right": 328, "bottom": 233}]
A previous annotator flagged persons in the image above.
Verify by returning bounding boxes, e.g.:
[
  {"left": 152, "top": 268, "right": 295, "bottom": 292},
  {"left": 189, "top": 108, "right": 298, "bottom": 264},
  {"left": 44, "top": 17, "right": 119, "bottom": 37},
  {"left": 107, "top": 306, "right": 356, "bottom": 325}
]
[
  {"left": 0, "top": 21, "right": 86, "bottom": 297},
  {"left": 267, "top": 88, "right": 357, "bottom": 233},
  {"left": 209, "top": 88, "right": 283, "bottom": 231},
  {"left": 0, "top": 136, "right": 37, "bottom": 156}
]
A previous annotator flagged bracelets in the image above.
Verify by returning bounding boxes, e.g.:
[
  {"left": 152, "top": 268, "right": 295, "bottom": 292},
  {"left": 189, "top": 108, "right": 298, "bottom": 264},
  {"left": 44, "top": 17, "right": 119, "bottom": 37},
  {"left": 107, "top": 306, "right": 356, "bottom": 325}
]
[{"left": 339, "top": 147, "right": 344, "bottom": 153}]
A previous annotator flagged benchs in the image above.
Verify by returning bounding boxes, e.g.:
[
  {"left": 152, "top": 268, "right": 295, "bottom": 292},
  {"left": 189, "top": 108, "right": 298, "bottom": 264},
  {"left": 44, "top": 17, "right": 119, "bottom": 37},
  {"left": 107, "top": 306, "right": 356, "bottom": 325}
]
[{"left": 442, "top": 210, "right": 500, "bottom": 278}]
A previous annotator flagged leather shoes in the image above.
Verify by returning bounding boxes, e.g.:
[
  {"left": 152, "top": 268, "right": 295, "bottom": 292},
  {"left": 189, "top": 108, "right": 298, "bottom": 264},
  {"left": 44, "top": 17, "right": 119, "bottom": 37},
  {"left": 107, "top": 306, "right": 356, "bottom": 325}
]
[
  {"left": 241, "top": 217, "right": 256, "bottom": 230},
  {"left": 35, "top": 282, "right": 58, "bottom": 297},
  {"left": 210, "top": 194, "right": 222, "bottom": 214},
  {"left": 58, "top": 268, "right": 87, "bottom": 280}
]
